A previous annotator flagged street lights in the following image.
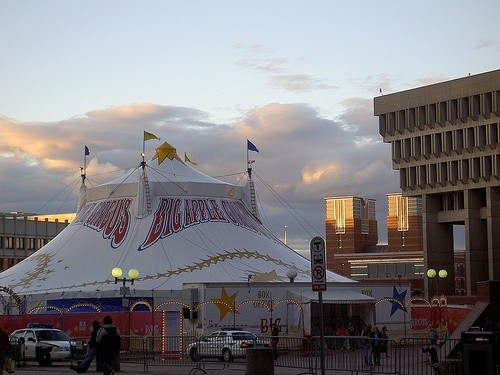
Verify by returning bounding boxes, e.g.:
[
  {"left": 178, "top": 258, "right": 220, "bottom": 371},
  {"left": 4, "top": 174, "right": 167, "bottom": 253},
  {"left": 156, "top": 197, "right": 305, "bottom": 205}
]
[
  {"left": 112, "top": 267, "right": 139, "bottom": 311},
  {"left": 426, "top": 269, "right": 447, "bottom": 296}
]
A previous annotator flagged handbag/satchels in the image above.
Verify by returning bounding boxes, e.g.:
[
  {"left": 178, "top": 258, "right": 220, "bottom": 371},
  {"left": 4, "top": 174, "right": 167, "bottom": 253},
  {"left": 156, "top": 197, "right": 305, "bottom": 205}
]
[{"left": 5, "top": 353, "right": 15, "bottom": 373}]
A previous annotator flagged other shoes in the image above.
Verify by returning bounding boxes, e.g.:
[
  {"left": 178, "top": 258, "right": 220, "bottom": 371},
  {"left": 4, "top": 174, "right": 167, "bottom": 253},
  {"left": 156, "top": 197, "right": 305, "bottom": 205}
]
[
  {"left": 374, "top": 362, "right": 381, "bottom": 366},
  {"left": 74, "top": 366, "right": 86, "bottom": 373},
  {"left": 369, "top": 362, "right": 373, "bottom": 366}
]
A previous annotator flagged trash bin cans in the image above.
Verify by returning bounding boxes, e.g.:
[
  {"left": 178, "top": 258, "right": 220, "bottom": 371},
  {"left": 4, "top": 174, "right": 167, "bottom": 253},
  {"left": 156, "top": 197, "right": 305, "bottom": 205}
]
[
  {"left": 245, "top": 347, "right": 275, "bottom": 375},
  {"left": 460, "top": 330, "right": 500, "bottom": 375}
]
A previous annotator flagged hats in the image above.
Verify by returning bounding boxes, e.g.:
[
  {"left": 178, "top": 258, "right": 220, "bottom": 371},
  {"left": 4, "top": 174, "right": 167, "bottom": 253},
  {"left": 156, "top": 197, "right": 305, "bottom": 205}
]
[{"left": 103, "top": 316, "right": 112, "bottom": 324}]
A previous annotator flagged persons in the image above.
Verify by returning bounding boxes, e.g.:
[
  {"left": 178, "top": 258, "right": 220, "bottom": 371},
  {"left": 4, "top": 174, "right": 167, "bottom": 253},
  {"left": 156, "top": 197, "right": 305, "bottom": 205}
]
[
  {"left": 360, "top": 324, "right": 373, "bottom": 366},
  {"left": 369, "top": 326, "right": 383, "bottom": 366},
  {"left": 380, "top": 326, "right": 390, "bottom": 358},
  {"left": 271, "top": 324, "right": 279, "bottom": 360},
  {"left": 69, "top": 320, "right": 102, "bottom": 374},
  {"left": 96, "top": 316, "right": 121, "bottom": 375},
  {"left": 429, "top": 328, "right": 438, "bottom": 346},
  {"left": 334, "top": 322, "right": 375, "bottom": 351},
  {"left": 0, "top": 326, "right": 12, "bottom": 375}
]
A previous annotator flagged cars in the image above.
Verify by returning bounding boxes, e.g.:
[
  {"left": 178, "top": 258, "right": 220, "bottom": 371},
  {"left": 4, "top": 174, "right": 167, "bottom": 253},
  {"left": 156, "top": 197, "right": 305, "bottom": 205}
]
[
  {"left": 8, "top": 328, "right": 88, "bottom": 359},
  {"left": 187, "top": 331, "right": 271, "bottom": 363}
]
